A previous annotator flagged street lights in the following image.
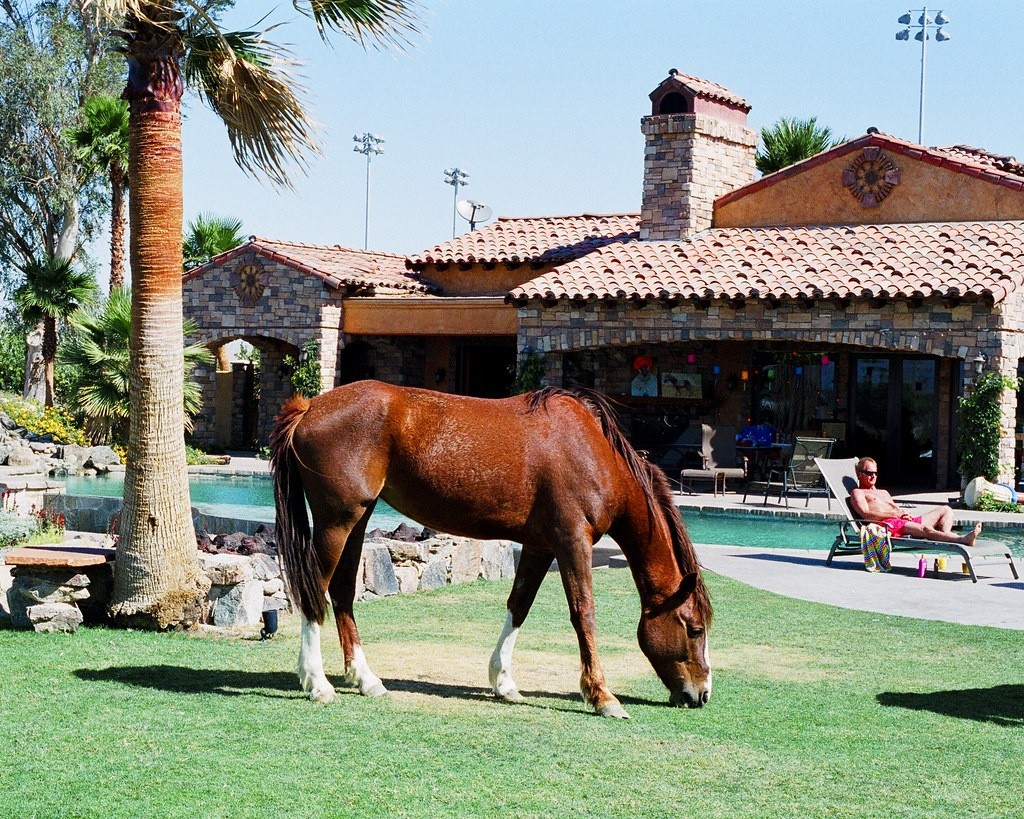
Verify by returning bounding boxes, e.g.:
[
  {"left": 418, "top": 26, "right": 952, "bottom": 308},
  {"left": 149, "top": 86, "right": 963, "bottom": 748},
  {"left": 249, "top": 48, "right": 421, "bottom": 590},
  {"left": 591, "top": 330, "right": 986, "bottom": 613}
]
[
  {"left": 352, "top": 131, "right": 388, "bottom": 251},
  {"left": 895, "top": 6, "right": 953, "bottom": 146},
  {"left": 444, "top": 166, "right": 470, "bottom": 239}
]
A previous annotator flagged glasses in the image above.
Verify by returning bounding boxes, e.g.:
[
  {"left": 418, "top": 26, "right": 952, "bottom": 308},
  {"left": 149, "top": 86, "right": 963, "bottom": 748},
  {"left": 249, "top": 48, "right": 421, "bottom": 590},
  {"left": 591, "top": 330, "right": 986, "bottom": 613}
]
[{"left": 859, "top": 469, "right": 878, "bottom": 476}]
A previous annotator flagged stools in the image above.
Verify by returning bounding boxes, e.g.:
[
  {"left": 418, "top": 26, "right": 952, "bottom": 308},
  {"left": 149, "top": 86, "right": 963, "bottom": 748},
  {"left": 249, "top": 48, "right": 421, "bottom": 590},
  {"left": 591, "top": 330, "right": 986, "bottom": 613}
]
[{"left": 680, "top": 469, "right": 725, "bottom": 498}]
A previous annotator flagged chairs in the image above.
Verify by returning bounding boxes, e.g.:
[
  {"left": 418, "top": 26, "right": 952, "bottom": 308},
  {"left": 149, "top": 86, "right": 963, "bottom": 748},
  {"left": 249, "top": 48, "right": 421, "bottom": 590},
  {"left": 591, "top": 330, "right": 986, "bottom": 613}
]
[
  {"left": 698, "top": 423, "right": 749, "bottom": 493},
  {"left": 743, "top": 436, "right": 836, "bottom": 510},
  {"left": 811, "top": 457, "right": 1019, "bottom": 584}
]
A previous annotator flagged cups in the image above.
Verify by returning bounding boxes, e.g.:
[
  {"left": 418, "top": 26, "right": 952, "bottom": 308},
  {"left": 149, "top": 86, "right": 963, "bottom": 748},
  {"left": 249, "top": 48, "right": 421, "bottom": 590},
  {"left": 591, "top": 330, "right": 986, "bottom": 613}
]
[
  {"left": 962, "top": 563, "right": 970, "bottom": 572},
  {"left": 939, "top": 554, "right": 947, "bottom": 570}
]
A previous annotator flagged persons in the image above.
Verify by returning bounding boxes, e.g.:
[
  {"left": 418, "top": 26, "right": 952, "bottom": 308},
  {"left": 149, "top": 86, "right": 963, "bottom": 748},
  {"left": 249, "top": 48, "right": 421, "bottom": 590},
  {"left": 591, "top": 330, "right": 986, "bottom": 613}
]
[
  {"left": 630, "top": 355, "right": 658, "bottom": 397},
  {"left": 850, "top": 457, "right": 982, "bottom": 547}
]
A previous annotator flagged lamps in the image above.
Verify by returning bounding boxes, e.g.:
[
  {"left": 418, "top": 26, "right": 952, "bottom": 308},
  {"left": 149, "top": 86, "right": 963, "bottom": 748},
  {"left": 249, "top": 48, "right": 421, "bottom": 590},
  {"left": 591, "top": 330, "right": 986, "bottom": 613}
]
[{"left": 742, "top": 364, "right": 750, "bottom": 393}]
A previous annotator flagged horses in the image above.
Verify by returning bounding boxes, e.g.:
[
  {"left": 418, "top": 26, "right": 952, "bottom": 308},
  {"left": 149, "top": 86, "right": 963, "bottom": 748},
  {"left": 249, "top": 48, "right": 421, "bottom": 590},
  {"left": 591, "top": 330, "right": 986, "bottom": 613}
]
[{"left": 267, "top": 379, "right": 713, "bottom": 721}]
